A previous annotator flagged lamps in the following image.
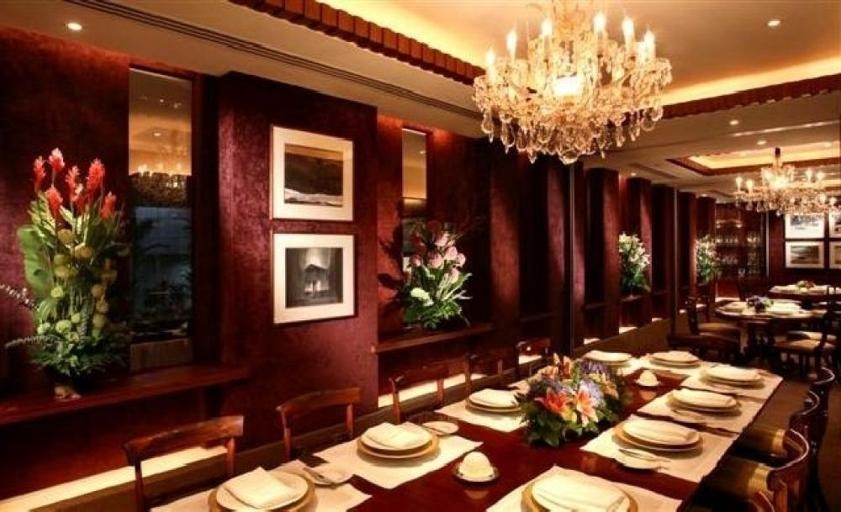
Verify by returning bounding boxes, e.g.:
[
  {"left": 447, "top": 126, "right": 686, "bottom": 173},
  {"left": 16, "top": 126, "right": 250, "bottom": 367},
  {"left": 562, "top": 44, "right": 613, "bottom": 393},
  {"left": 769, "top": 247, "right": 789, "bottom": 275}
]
[
  {"left": 472, "top": 0, "right": 673, "bottom": 164},
  {"left": 734, "top": 148, "right": 839, "bottom": 220}
]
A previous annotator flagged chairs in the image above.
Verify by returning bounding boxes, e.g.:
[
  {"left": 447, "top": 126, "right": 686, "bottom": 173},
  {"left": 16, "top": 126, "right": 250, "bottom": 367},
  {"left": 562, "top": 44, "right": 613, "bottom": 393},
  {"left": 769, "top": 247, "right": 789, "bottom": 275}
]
[
  {"left": 389, "top": 363, "right": 449, "bottom": 421},
  {"left": 717, "top": 428, "right": 810, "bottom": 512},
  {"left": 747, "top": 490, "right": 775, "bottom": 512},
  {"left": 274, "top": 387, "right": 361, "bottom": 464},
  {"left": 685, "top": 297, "right": 741, "bottom": 358},
  {"left": 746, "top": 389, "right": 821, "bottom": 458},
  {"left": 520, "top": 335, "right": 551, "bottom": 377},
  {"left": 460, "top": 347, "right": 511, "bottom": 397},
  {"left": 771, "top": 285, "right": 841, "bottom": 379},
  {"left": 810, "top": 365, "right": 836, "bottom": 445},
  {"left": 120, "top": 413, "right": 244, "bottom": 512}
]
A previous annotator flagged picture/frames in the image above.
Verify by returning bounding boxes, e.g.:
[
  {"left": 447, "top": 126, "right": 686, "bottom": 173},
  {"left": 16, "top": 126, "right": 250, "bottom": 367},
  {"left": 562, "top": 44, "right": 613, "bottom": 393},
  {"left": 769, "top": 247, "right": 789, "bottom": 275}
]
[
  {"left": 271, "top": 233, "right": 358, "bottom": 325},
  {"left": 828, "top": 241, "right": 841, "bottom": 270},
  {"left": 829, "top": 211, "right": 841, "bottom": 238},
  {"left": 784, "top": 213, "right": 825, "bottom": 239},
  {"left": 784, "top": 241, "right": 825, "bottom": 269},
  {"left": 269, "top": 125, "right": 354, "bottom": 224}
]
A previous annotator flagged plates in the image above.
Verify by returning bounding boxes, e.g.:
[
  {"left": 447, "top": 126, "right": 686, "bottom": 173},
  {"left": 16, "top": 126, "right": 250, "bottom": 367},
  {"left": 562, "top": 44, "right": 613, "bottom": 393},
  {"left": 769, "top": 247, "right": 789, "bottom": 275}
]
[
  {"left": 465, "top": 394, "right": 521, "bottom": 413},
  {"left": 653, "top": 352, "right": 698, "bottom": 363},
  {"left": 361, "top": 425, "right": 432, "bottom": 451},
  {"left": 649, "top": 354, "right": 701, "bottom": 367},
  {"left": 615, "top": 449, "right": 661, "bottom": 469},
  {"left": 615, "top": 419, "right": 703, "bottom": 453},
  {"left": 216, "top": 473, "right": 308, "bottom": 512},
  {"left": 357, "top": 428, "right": 438, "bottom": 460},
  {"left": 531, "top": 477, "right": 630, "bottom": 512},
  {"left": 634, "top": 379, "right": 661, "bottom": 388},
  {"left": 469, "top": 391, "right": 520, "bottom": 407},
  {"left": 305, "top": 462, "right": 354, "bottom": 485},
  {"left": 668, "top": 393, "right": 737, "bottom": 413},
  {"left": 707, "top": 366, "right": 761, "bottom": 382},
  {"left": 623, "top": 420, "right": 700, "bottom": 447},
  {"left": 671, "top": 410, "right": 709, "bottom": 424},
  {"left": 453, "top": 461, "right": 500, "bottom": 484},
  {"left": 584, "top": 353, "right": 630, "bottom": 366},
  {"left": 524, "top": 474, "right": 639, "bottom": 512},
  {"left": 707, "top": 374, "right": 764, "bottom": 387},
  {"left": 207, "top": 470, "right": 315, "bottom": 512},
  {"left": 422, "top": 421, "right": 458, "bottom": 436},
  {"left": 672, "top": 390, "right": 737, "bottom": 408}
]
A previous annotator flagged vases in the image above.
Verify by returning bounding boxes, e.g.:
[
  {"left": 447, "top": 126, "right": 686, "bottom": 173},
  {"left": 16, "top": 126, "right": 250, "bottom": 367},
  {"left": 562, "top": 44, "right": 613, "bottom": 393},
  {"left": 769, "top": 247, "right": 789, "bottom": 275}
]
[{"left": 56, "top": 369, "right": 88, "bottom": 399}]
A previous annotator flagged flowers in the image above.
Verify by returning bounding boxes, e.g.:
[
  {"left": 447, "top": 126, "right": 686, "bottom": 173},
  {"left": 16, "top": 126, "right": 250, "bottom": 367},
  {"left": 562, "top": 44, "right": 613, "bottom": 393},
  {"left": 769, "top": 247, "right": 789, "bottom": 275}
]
[
  {"left": 694, "top": 240, "right": 719, "bottom": 282},
  {"left": 511, "top": 352, "right": 621, "bottom": 446},
  {"left": 618, "top": 230, "right": 652, "bottom": 294},
  {"left": 400, "top": 220, "right": 474, "bottom": 329},
  {"left": 1, "top": 146, "right": 140, "bottom": 375},
  {"left": 746, "top": 296, "right": 773, "bottom": 314}
]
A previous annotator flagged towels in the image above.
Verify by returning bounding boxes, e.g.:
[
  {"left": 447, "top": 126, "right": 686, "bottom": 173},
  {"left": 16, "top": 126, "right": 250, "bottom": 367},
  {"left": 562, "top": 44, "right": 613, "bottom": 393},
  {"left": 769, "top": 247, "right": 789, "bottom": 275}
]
[
  {"left": 221, "top": 466, "right": 296, "bottom": 510},
  {"left": 366, "top": 422, "right": 426, "bottom": 448},
  {"left": 679, "top": 388, "right": 732, "bottom": 406},
  {"left": 472, "top": 388, "right": 513, "bottom": 406},
  {"left": 637, "top": 420, "right": 696, "bottom": 443},
  {"left": 538, "top": 474, "right": 624, "bottom": 512}
]
